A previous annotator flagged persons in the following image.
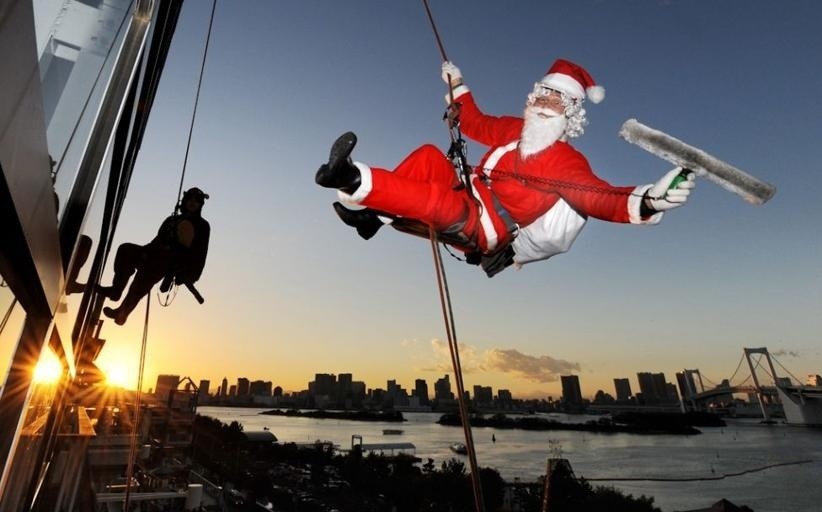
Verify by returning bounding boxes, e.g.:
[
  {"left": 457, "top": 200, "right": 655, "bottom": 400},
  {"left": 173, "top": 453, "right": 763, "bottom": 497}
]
[
  {"left": 314, "top": 58, "right": 695, "bottom": 277},
  {"left": 97, "top": 187, "right": 211, "bottom": 326}
]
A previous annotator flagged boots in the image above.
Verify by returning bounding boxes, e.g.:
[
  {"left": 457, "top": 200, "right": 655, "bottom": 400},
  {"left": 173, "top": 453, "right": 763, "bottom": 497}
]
[
  {"left": 315, "top": 132, "right": 361, "bottom": 195},
  {"left": 332, "top": 201, "right": 385, "bottom": 240}
]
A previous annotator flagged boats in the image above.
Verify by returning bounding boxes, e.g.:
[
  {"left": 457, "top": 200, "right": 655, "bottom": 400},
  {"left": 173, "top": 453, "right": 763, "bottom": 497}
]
[
  {"left": 449, "top": 440, "right": 468, "bottom": 456},
  {"left": 381, "top": 429, "right": 404, "bottom": 435}
]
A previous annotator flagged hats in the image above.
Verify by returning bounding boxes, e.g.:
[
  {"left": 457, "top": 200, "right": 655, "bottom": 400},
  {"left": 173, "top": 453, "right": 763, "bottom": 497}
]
[{"left": 539, "top": 58, "right": 606, "bottom": 104}]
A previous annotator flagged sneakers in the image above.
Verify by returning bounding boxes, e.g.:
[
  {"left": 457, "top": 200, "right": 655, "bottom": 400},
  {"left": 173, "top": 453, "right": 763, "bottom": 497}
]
[
  {"left": 103, "top": 306, "right": 128, "bottom": 326},
  {"left": 95, "top": 284, "right": 122, "bottom": 301}
]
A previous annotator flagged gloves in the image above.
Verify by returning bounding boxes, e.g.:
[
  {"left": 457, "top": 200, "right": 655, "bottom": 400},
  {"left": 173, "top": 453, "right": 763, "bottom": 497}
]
[
  {"left": 441, "top": 60, "right": 463, "bottom": 84},
  {"left": 647, "top": 166, "right": 696, "bottom": 211}
]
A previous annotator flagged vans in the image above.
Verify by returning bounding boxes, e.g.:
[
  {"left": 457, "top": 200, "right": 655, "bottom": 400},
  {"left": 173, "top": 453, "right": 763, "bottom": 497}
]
[{"left": 230, "top": 488, "right": 244, "bottom": 505}]
